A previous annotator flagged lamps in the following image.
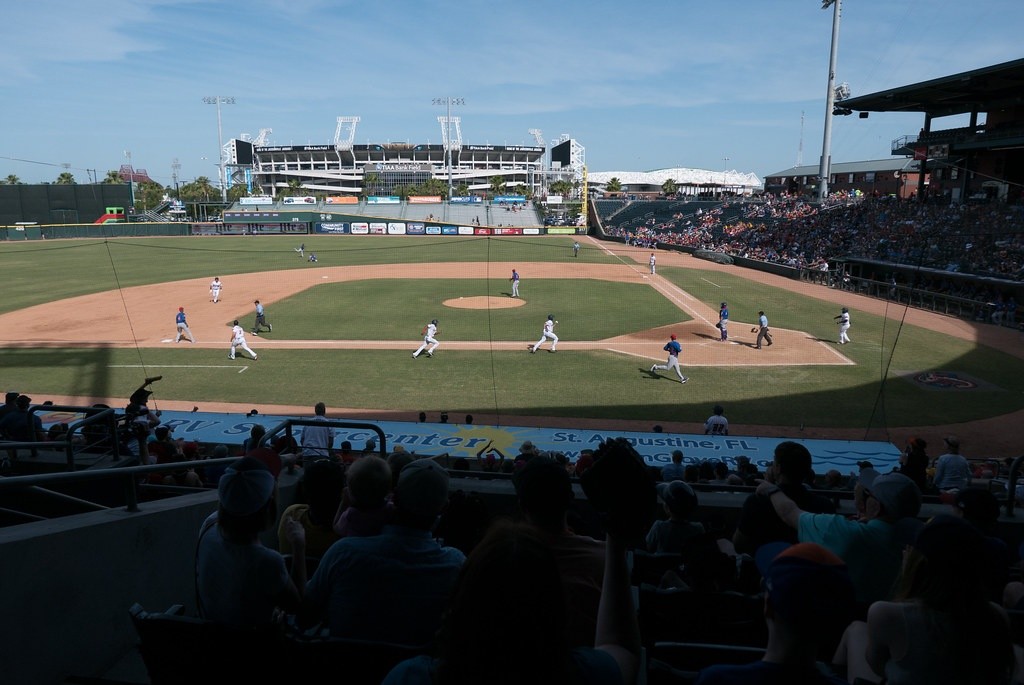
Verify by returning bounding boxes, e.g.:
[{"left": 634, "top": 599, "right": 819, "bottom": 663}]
[{"left": 832, "top": 106, "right": 869, "bottom": 119}]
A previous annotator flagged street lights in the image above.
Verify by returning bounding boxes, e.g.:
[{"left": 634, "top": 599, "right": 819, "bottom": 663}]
[
  {"left": 722, "top": 157, "right": 729, "bottom": 184},
  {"left": 432, "top": 96, "right": 466, "bottom": 202},
  {"left": 819, "top": 0, "right": 842, "bottom": 202},
  {"left": 676, "top": 165, "right": 682, "bottom": 183},
  {"left": 202, "top": 96, "right": 237, "bottom": 202}
]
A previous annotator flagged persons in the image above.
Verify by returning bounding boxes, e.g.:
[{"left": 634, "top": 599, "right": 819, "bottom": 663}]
[
  {"left": 228, "top": 320, "right": 257, "bottom": 361},
  {"left": 717, "top": 302, "right": 729, "bottom": 341},
  {"left": 175, "top": 307, "right": 195, "bottom": 343},
  {"left": 413, "top": 319, "right": 439, "bottom": 359},
  {"left": 834, "top": 308, "right": 850, "bottom": 344},
  {"left": 509, "top": 269, "right": 520, "bottom": 298},
  {"left": 241, "top": 207, "right": 248, "bottom": 211},
  {"left": 605, "top": 188, "right": 896, "bottom": 285},
  {"left": 649, "top": 253, "right": 656, "bottom": 275},
  {"left": 484, "top": 200, "right": 528, "bottom": 214},
  {"left": 193, "top": 403, "right": 1024, "bottom": 685},
  {"left": 0, "top": 377, "right": 229, "bottom": 487},
  {"left": 210, "top": 277, "right": 222, "bottom": 303},
  {"left": 830, "top": 157, "right": 1024, "bottom": 328},
  {"left": 754, "top": 311, "right": 773, "bottom": 349},
  {"left": 573, "top": 242, "right": 579, "bottom": 258},
  {"left": 531, "top": 314, "right": 559, "bottom": 354},
  {"left": 256, "top": 205, "right": 258, "bottom": 211},
  {"left": 426, "top": 213, "right": 513, "bottom": 228},
  {"left": 292, "top": 244, "right": 305, "bottom": 257},
  {"left": 251, "top": 300, "right": 272, "bottom": 336},
  {"left": 308, "top": 254, "right": 317, "bottom": 262},
  {"left": 651, "top": 334, "right": 689, "bottom": 383}
]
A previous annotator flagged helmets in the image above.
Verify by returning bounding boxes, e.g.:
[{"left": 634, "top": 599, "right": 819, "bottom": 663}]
[
  {"left": 840, "top": 308, "right": 848, "bottom": 312},
  {"left": 548, "top": 314, "right": 555, "bottom": 321},
  {"left": 721, "top": 300, "right": 727, "bottom": 305},
  {"left": 432, "top": 320, "right": 437, "bottom": 326}
]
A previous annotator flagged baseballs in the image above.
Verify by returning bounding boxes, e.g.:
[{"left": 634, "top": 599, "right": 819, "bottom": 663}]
[{"left": 556, "top": 321, "right": 558, "bottom": 323}]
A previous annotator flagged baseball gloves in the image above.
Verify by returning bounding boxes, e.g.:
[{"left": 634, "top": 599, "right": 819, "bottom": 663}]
[
  {"left": 751, "top": 328, "right": 758, "bottom": 333},
  {"left": 716, "top": 323, "right": 721, "bottom": 328}
]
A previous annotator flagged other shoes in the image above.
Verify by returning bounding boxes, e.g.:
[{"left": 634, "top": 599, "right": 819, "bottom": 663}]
[
  {"left": 754, "top": 342, "right": 772, "bottom": 349},
  {"left": 650, "top": 363, "right": 658, "bottom": 372},
  {"left": 837, "top": 339, "right": 850, "bottom": 345},
  {"left": 510, "top": 293, "right": 520, "bottom": 298},
  {"left": 681, "top": 376, "right": 690, "bottom": 384},
  {"left": 252, "top": 324, "right": 272, "bottom": 336},
  {"left": 412, "top": 352, "right": 434, "bottom": 362},
  {"left": 228, "top": 355, "right": 258, "bottom": 360}
]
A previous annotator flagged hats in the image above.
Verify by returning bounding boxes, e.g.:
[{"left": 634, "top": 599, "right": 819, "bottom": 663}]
[
  {"left": 519, "top": 441, "right": 536, "bottom": 454},
  {"left": 48, "top": 423, "right": 68, "bottom": 441},
  {"left": 130, "top": 387, "right": 153, "bottom": 399},
  {"left": 218, "top": 455, "right": 275, "bottom": 517},
  {"left": 655, "top": 480, "right": 698, "bottom": 511},
  {"left": 856, "top": 462, "right": 982, "bottom": 571},
  {"left": 6, "top": 392, "right": 31, "bottom": 404},
  {"left": 671, "top": 333, "right": 677, "bottom": 339},
  {"left": 911, "top": 437, "right": 959, "bottom": 450},
  {"left": 396, "top": 458, "right": 451, "bottom": 514},
  {"left": 757, "top": 311, "right": 764, "bottom": 316}
]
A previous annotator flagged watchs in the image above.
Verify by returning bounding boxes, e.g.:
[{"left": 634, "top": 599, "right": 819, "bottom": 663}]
[{"left": 767, "top": 487, "right": 781, "bottom": 499}]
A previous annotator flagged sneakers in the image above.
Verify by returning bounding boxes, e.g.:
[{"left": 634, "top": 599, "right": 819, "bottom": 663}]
[{"left": 533, "top": 347, "right": 558, "bottom": 354}]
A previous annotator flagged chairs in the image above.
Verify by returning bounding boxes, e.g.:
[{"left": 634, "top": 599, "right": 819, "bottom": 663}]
[{"left": 130, "top": 554, "right": 843, "bottom": 684}]
